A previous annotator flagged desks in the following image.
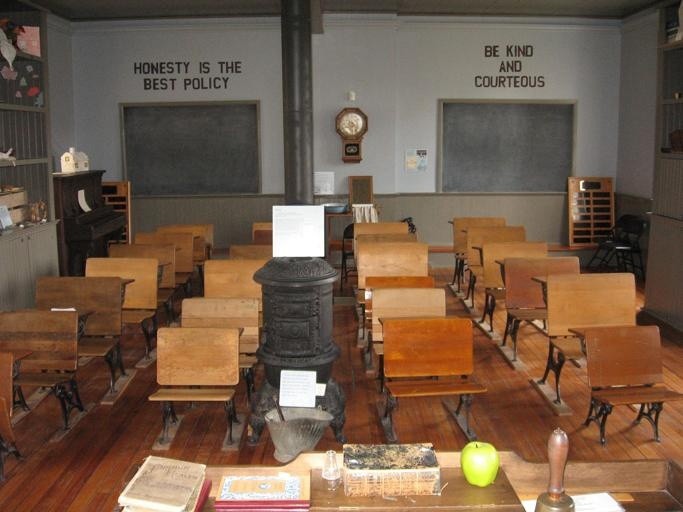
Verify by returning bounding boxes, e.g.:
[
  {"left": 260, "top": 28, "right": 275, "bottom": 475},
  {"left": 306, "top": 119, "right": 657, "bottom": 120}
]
[
  {"left": 309, "top": 466, "right": 526, "bottom": 511},
  {"left": 325, "top": 211, "right": 380, "bottom": 262}
]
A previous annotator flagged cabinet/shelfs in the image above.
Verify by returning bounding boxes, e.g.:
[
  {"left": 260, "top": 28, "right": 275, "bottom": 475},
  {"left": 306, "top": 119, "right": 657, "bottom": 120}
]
[
  {"left": 0, "top": 0, "right": 61, "bottom": 310},
  {"left": 643, "top": 0, "right": 683, "bottom": 332}
]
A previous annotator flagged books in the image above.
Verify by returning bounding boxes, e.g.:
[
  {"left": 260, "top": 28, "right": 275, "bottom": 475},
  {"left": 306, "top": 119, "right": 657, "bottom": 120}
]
[
  {"left": 342, "top": 443, "right": 442, "bottom": 496},
  {"left": 118, "top": 455, "right": 213, "bottom": 512},
  {"left": 214, "top": 469, "right": 312, "bottom": 512}
]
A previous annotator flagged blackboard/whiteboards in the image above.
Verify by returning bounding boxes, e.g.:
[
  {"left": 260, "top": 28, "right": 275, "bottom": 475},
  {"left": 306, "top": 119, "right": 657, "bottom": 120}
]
[
  {"left": 436, "top": 99, "right": 579, "bottom": 195},
  {"left": 119, "top": 100, "right": 263, "bottom": 198}
]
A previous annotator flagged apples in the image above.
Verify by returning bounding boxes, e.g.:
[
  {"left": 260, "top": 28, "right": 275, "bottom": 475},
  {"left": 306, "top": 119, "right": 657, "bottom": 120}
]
[{"left": 461, "top": 441, "right": 500, "bottom": 487}]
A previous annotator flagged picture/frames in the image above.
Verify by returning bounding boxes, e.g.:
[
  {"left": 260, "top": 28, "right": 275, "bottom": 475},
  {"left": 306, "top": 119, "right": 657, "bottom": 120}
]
[{"left": 348, "top": 175, "right": 374, "bottom": 208}]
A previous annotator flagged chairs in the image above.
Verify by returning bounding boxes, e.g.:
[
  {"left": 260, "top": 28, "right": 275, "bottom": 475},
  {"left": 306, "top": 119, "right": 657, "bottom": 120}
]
[
  {"left": 149, "top": 326, "right": 241, "bottom": 447},
  {"left": 181, "top": 298, "right": 258, "bottom": 412},
  {"left": 3, "top": 309, "right": 85, "bottom": 430},
  {"left": 582, "top": 325, "right": 682, "bottom": 448},
  {"left": 370, "top": 287, "right": 447, "bottom": 391},
  {"left": 86, "top": 223, "right": 580, "bottom": 361},
  {"left": 2, "top": 352, "right": 24, "bottom": 482},
  {"left": 376, "top": 318, "right": 492, "bottom": 451},
  {"left": 33, "top": 275, "right": 129, "bottom": 397},
  {"left": 535, "top": 271, "right": 635, "bottom": 404}
]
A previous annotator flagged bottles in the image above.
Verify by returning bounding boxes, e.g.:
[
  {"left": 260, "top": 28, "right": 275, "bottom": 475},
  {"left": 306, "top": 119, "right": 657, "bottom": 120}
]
[{"left": 322, "top": 450, "right": 338, "bottom": 480}]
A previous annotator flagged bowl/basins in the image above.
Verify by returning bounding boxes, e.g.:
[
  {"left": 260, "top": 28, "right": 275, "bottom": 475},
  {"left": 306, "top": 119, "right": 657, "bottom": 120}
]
[{"left": 322, "top": 203, "right": 348, "bottom": 213}]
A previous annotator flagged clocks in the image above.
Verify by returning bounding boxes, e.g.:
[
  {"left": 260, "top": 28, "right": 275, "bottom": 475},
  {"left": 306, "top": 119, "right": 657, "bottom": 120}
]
[{"left": 335, "top": 107, "right": 368, "bottom": 162}]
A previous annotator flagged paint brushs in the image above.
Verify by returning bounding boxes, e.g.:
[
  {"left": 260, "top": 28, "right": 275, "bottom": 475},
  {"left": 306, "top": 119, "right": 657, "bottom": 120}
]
[{"left": 272, "top": 394, "right": 284, "bottom": 422}]
